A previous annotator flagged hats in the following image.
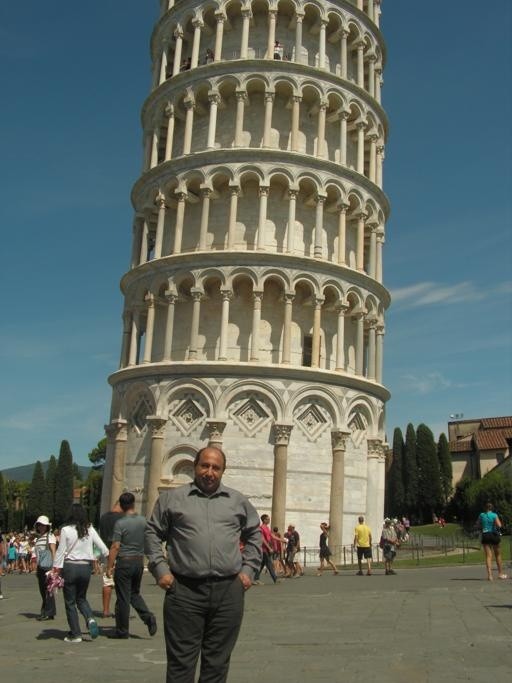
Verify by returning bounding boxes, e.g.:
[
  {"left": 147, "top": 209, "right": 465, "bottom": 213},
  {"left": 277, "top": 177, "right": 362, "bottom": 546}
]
[{"left": 34, "top": 515, "right": 52, "bottom": 534}]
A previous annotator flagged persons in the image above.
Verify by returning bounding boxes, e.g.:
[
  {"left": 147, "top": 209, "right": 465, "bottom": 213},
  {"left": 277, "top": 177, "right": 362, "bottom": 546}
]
[
  {"left": 0, "top": 492, "right": 157, "bottom": 643},
  {"left": 238, "top": 514, "right": 412, "bottom": 585},
  {"left": 439, "top": 515, "right": 446, "bottom": 527},
  {"left": 474, "top": 503, "right": 508, "bottom": 580},
  {"left": 142, "top": 445, "right": 263, "bottom": 683},
  {"left": 432, "top": 513, "right": 439, "bottom": 527}
]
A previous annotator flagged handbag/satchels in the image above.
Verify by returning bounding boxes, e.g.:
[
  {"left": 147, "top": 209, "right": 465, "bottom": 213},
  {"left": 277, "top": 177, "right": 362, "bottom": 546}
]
[{"left": 384, "top": 545, "right": 390, "bottom": 554}]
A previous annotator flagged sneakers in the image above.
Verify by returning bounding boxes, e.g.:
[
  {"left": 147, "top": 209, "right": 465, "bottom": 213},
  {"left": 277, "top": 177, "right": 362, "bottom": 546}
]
[
  {"left": 385, "top": 569, "right": 396, "bottom": 575},
  {"left": 146, "top": 615, "right": 157, "bottom": 636},
  {"left": 64, "top": 636, "right": 81, "bottom": 642},
  {"left": 106, "top": 632, "right": 128, "bottom": 639},
  {"left": 356, "top": 571, "right": 372, "bottom": 575},
  {"left": 87, "top": 619, "right": 98, "bottom": 639}
]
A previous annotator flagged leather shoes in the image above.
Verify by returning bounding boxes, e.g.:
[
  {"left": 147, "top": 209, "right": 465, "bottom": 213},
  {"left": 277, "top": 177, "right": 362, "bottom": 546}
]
[{"left": 36, "top": 615, "right": 54, "bottom": 620}]
